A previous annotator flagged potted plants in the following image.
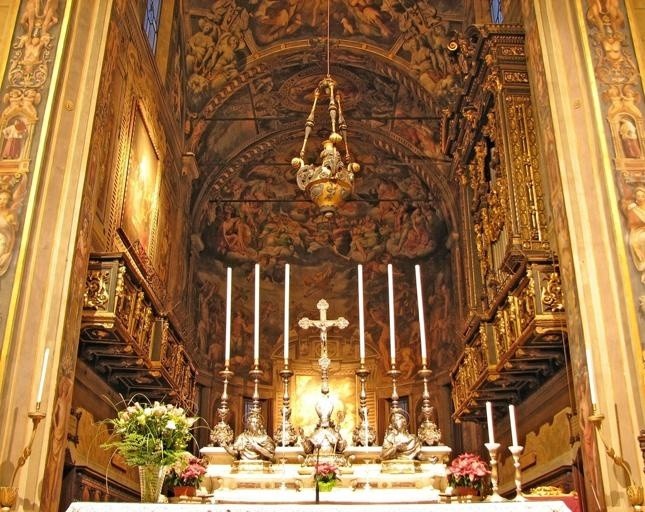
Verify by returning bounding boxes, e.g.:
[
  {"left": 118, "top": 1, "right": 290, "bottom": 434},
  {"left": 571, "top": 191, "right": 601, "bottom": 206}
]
[{"left": 388, "top": 264, "right": 395, "bottom": 358}]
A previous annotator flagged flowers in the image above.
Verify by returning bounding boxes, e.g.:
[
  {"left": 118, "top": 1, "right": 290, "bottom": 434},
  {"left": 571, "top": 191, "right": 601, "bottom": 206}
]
[
  {"left": 164, "top": 456, "right": 208, "bottom": 489},
  {"left": 449, "top": 451, "right": 491, "bottom": 488},
  {"left": 314, "top": 462, "right": 341, "bottom": 483},
  {"left": 86, "top": 391, "right": 212, "bottom": 495}
]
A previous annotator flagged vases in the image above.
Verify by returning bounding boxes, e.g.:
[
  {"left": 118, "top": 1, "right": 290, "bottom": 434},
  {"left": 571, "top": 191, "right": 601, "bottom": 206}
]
[
  {"left": 172, "top": 484, "right": 196, "bottom": 495},
  {"left": 138, "top": 462, "right": 168, "bottom": 502},
  {"left": 317, "top": 480, "right": 334, "bottom": 491},
  {"left": 456, "top": 486, "right": 478, "bottom": 494}
]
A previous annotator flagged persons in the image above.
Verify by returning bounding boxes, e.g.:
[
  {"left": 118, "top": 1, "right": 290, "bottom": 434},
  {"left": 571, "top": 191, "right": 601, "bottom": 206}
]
[
  {"left": 110, "top": 1, "right": 512, "bottom": 460},
  {"left": 0, "top": 1, "right": 62, "bottom": 279},
  {"left": 582, "top": 1, "right": 645, "bottom": 356}
]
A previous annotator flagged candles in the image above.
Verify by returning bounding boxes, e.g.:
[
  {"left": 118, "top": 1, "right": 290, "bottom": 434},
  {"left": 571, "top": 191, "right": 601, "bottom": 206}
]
[
  {"left": 414, "top": 264, "right": 426, "bottom": 356},
  {"left": 36, "top": 347, "right": 49, "bottom": 400},
  {"left": 357, "top": 264, "right": 365, "bottom": 358},
  {"left": 225, "top": 267, "right": 232, "bottom": 360},
  {"left": 585, "top": 348, "right": 598, "bottom": 403},
  {"left": 486, "top": 401, "right": 495, "bottom": 442},
  {"left": 254, "top": 263, "right": 260, "bottom": 359},
  {"left": 284, "top": 263, "right": 290, "bottom": 358},
  {"left": 507, "top": 404, "right": 519, "bottom": 446}
]
